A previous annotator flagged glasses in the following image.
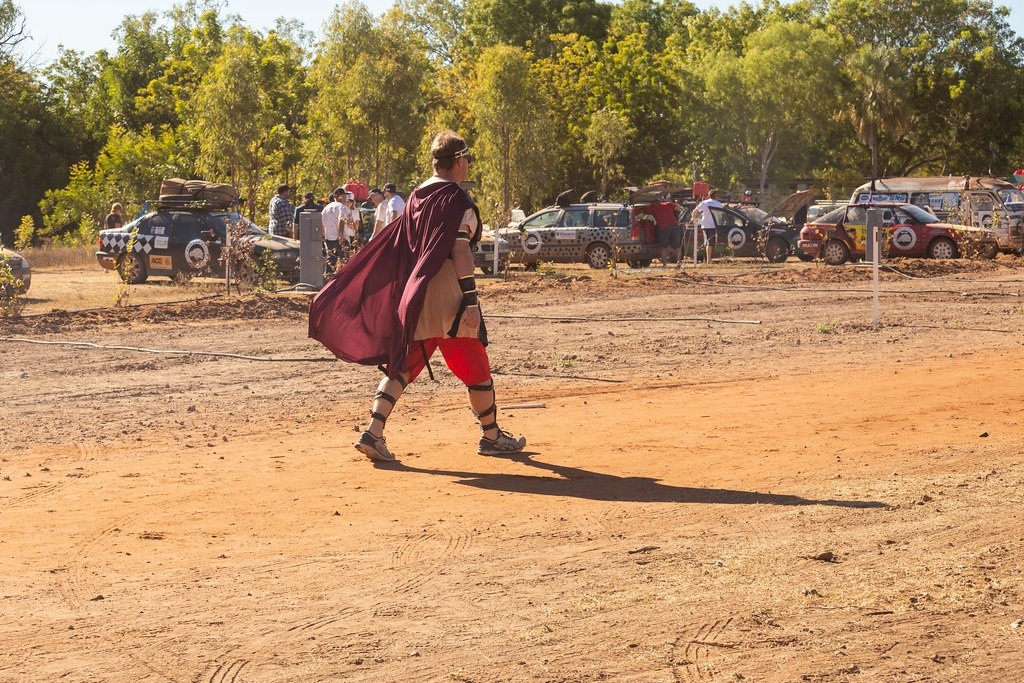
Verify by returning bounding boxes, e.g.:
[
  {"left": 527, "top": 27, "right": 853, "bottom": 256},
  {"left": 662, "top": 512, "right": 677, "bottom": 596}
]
[{"left": 463, "top": 156, "right": 472, "bottom": 164}]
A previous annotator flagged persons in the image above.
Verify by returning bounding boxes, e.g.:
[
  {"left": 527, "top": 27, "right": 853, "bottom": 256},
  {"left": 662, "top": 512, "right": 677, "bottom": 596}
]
[
  {"left": 354, "top": 129, "right": 525, "bottom": 463},
  {"left": 691, "top": 188, "right": 726, "bottom": 263},
  {"left": 578, "top": 212, "right": 589, "bottom": 228},
  {"left": 642, "top": 197, "right": 681, "bottom": 268},
  {"left": 104, "top": 202, "right": 122, "bottom": 273},
  {"left": 268, "top": 183, "right": 407, "bottom": 272}
]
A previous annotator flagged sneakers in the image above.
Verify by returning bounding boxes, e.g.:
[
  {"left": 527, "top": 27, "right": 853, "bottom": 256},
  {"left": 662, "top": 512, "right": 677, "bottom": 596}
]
[
  {"left": 478, "top": 428, "right": 527, "bottom": 454},
  {"left": 356, "top": 432, "right": 395, "bottom": 462}
]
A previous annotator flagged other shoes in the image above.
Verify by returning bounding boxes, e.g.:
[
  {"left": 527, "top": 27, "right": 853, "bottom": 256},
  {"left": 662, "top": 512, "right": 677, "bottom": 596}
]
[{"left": 677, "top": 261, "right": 681, "bottom": 267}]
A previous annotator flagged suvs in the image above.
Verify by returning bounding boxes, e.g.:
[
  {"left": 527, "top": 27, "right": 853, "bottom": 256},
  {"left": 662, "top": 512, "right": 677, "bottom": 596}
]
[{"left": 487, "top": 179, "right": 816, "bottom": 271}]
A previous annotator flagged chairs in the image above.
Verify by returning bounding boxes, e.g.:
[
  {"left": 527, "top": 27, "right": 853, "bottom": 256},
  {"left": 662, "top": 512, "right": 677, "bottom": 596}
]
[{"left": 883, "top": 211, "right": 893, "bottom": 227}]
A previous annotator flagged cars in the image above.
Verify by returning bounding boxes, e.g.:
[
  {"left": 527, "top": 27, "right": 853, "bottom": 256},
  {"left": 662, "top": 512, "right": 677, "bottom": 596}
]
[
  {"left": 354, "top": 204, "right": 510, "bottom": 276},
  {"left": 796, "top": 202, "right": 997, "bottom": 266},
  {"left": 96, "top": 206, "right": 300, "bottom": 285},
  {"left": 0, "top": 247, "right": 31, "bottom": 295}
]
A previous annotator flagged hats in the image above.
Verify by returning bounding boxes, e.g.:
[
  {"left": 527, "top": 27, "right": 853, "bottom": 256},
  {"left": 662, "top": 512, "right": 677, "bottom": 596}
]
[
  {"left": 346, "top": 191, "right": 354, "bottom": 200},
  {"left": 744, "top": 190, "right": 752, "bottom": 196},
  {"left": 380, "top": 183, "right": 396, "bottom": 191},
  {"left": 367, "top": 188, "right": 381, "bottom": 200},
  {"left": 333, "top": 188, "right": 351, "bottom": 197}
]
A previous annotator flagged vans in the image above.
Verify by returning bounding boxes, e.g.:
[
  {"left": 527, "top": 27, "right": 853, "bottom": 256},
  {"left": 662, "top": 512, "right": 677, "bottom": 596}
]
[
  {"left": 807, "top": 198, "right": 851, "bottom": 223},
  {"left": 848, "top": 175, "right": 1024, "bottom": 260}
]
